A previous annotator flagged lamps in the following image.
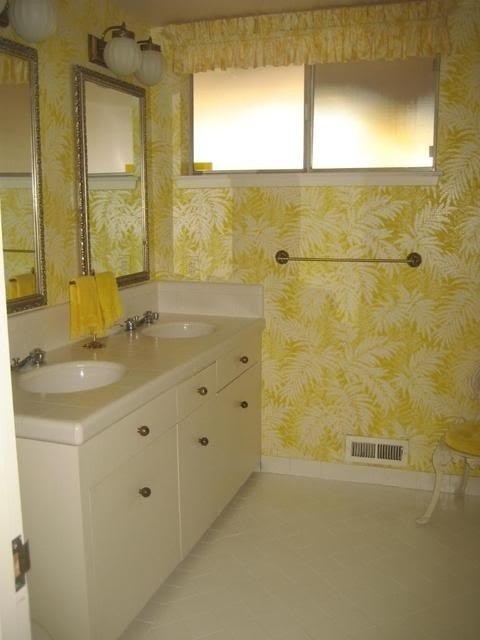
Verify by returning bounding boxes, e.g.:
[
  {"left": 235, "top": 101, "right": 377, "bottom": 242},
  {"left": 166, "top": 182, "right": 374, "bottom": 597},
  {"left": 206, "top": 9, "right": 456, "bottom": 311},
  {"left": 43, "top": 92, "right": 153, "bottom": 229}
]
[
  {"left": 1, "top": 0, "right": 58, "bottom": 44},
  {"left": 88, "top": 21, "right": 167, "bottom": 87}
]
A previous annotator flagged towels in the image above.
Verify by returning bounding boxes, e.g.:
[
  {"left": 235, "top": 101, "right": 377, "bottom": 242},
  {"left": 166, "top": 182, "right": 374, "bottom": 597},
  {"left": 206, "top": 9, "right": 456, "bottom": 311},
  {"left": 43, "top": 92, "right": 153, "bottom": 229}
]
[
  {"left": 6, "top": 273, "right": 35, "bottom": 302},
  {"left": 70, "top": 270, "right": 124, "bottom": 335}
]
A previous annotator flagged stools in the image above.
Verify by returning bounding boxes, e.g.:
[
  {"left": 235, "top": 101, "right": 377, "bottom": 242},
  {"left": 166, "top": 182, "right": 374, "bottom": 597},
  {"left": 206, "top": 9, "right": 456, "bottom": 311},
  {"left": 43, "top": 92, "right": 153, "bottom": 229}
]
[{"left": 415, "top": 426, "right": 479, "bottom": 525}]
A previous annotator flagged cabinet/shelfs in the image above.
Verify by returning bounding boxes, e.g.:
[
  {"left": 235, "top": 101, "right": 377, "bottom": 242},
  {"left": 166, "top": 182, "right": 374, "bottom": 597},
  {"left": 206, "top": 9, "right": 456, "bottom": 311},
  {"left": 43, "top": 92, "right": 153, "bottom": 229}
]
[{"left": 16, "top": 332, "right": 263, "bottom": 639}]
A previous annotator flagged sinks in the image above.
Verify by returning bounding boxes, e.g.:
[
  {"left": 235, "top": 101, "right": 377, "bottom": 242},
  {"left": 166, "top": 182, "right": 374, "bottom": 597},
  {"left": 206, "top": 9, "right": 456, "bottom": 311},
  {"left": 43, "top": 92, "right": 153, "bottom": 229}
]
[
  {"left": 142, "top": 320, "right": 212, "bottom": 339},
  {"left": 16, "top": 359, "right": 125, "bottom": 394}
]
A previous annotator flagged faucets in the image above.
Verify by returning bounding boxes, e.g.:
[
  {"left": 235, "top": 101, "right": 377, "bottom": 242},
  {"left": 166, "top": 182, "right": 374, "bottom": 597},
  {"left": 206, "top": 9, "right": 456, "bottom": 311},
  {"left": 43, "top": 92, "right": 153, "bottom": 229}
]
[
  {"left": 11, "top": 352, "right": 45, "bottom": 373},
  {"left": 125, "top": 311, "right": 160, "bottom": 331}
]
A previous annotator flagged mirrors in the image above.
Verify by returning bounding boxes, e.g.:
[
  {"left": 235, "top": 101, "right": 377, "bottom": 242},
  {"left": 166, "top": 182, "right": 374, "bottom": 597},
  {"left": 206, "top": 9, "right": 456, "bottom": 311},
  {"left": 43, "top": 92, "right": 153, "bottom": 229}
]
[
  {"left": 0, "top": 37, "right": 48, "bottom": 320},
  {"left": 72, "top": 64, "right": 150, "bottom": 291}
]
